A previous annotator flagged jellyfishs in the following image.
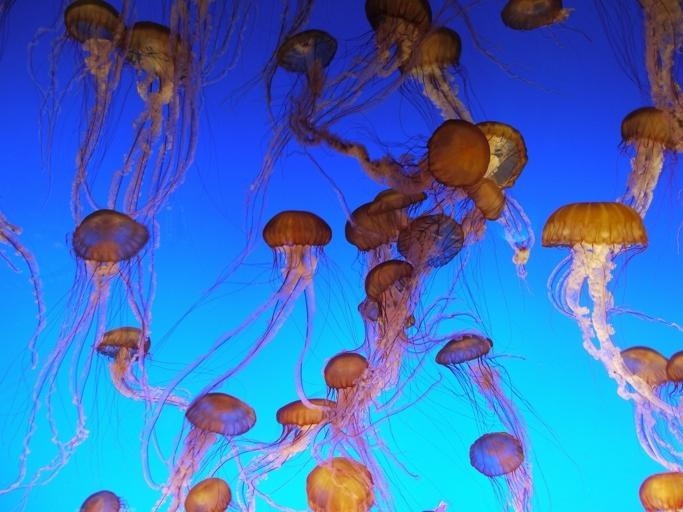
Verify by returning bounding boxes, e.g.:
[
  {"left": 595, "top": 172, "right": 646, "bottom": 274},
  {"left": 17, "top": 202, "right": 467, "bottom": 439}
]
[{"left": 0, "top": 0, "right": 683, "bottom": 512}]
